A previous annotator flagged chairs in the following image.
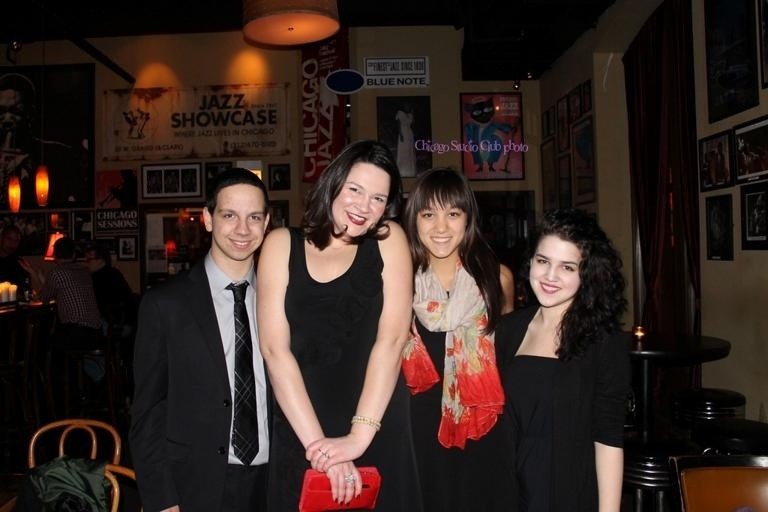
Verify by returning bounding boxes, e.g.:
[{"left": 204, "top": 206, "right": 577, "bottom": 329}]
[
  {"left": 0, "top": 319, "right": 41, "bottom": 454},
  {"left": 25, "top": 459, "right": 144, "bottom": 512},
  {"left": 50, "top": 314, "right": 124, "bottom": 426},
  {"left": 28, "top": 419, "right": 122, "bottom": 468}
]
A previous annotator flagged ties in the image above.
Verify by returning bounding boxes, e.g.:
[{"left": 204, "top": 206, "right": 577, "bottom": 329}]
[{"left": 225, "top": 281, "right": 259, "bottom": 465}]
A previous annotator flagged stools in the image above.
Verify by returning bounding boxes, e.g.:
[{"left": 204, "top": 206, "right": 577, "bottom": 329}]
[
  {"left": 669, "top": 387, "right": 746, "bottom": 435},
  {"left": 690, "top": 417, "right": 768, "bottom": 466},
  {"left": 623, "top": 451, "right": 675, "bottom": 512}
]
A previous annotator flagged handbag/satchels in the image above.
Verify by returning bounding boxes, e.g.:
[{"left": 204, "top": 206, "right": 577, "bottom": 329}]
[{"left": 299, "top": 467, "right": 382, "bottom": 511}]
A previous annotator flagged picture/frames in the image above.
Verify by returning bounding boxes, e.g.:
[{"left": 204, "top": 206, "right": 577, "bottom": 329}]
[
  {"left": 699, "top": 128, "right": 735, "bottom": 192},
  {"left": 740, "top": 181, "right": 768, "bottom": 251},
  {"left": 732, "top": 113, "right": 768, "bottom": 185},
  {"left": 117, "top": 235, "right": 138, "bottom": 261},
  {"left": 204, "top": 161, "right": 232, "bottom": 183},
  {"left": 757, "top": 0, "right": 768, "bottom": 90},
  {"left": 540, "top": 79, "right": 596, "bottom": 211},
  {"left": 705, "top": 194, "right": 734, "bottom": 261},
  {"left": 459, "top": 91, "right": 525, "bottom": 181},
  {"left": 141, "top": 162, "right": 203, "bottom": 200},
  {"left": 704, "top": 0, "right": 760, "bottom": 124}
]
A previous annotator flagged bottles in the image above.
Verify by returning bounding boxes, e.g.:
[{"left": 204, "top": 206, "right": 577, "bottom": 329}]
[{"left": 21, "top": 276, "right": 32, "bottom": 302}]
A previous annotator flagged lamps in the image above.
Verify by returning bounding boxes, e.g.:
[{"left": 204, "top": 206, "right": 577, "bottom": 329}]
[
  {"left": 242, "top": 0, "right": 341, "bottom": 51},
  {"left": 7, "top": 38, "right": 22, "bottom": 214},
  {"left": 35, "top": 43, "right": 49, "bottom": 208}
]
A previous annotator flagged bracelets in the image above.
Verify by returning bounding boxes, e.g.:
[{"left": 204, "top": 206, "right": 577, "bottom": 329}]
[{"left": 351, "top": 416, "right": 381, "bottom": 432}]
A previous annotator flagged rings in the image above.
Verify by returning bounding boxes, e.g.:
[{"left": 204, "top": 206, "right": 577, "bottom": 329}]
[
  {"left": 345, "top": 474, "right": 357, "bottom": 485},
  {"left": 324, "top": 453, "right": 330, "bottom": 459},
  {"left": 318, "top": 449, "right": 324, "bottom": 454}
]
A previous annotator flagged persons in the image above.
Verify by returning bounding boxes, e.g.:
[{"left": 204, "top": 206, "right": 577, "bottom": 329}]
[
  {"left": 393, "top": 102, "right": 416, "bottom": 178},
  {"left": 495, "top": 207, "right": 632, "bottom": 512},
  {"left": 401, "top": 167, "right": 522, "bottom": 511},
  {"left": 258, "top": 140, "right": 425, "bottom": 512},
  {"left": 128, "top": 168, "right": 272, "bottom": 512},
  {"left": 0, "top": 226, "right": 27, "bottom": 303},
  {"left": 31, "top": 238, "right": 135, "bottom": 384}
]
